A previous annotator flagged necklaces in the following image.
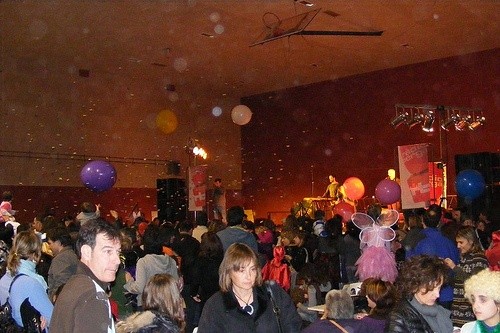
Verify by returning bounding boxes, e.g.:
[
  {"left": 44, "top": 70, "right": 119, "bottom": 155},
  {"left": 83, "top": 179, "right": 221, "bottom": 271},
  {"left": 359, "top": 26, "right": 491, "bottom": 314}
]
[{"left": 234, "top": 292, "right": 254, "bottom": 315}]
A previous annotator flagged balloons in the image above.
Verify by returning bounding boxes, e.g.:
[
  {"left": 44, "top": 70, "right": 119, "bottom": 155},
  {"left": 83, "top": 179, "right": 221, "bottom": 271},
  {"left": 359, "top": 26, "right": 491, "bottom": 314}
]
[
  {"left": 455, "top": 168, "right": 486, "bottom": 202},
  {"left": 332, "top": 202, "right": 354, "bottom": 222},
  {"left": 80, "top": 159, "right": 117, "bottom": 192},
  {"left": 231, "top": 105, "right": 252, "bottom": 125},
  {"left": 375, "top": 180, "right": 400, "bottom": 205},
  {"left": 156, "top": 109, "right": 178, "bottom": 134},
  {"left": 343, "top": 177, "right": 365, "bottom": 201}
]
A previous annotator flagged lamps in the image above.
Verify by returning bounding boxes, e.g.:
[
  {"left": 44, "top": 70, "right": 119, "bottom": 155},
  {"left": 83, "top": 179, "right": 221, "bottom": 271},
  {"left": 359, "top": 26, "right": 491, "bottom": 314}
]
[
  {"left": 391, "top": 104, "right": 486, "bottom": 130},
  {"left": 186, "top": 138, "right": 207, "bottom": 161}
]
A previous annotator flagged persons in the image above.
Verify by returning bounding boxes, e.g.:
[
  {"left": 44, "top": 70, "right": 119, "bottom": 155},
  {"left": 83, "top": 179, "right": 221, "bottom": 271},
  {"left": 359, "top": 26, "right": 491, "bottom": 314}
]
[
  {"left": 211, "top": 178, "right": 228, "bottom": 226},
  {"left": 322, "top": 174, "right": 339, "bottom": 217},
  {"left": 0, "top": 202, "right": 500, "bottom": 333},
  {"left": 385, "top": 169, "right": 401, "bottom": 211}
]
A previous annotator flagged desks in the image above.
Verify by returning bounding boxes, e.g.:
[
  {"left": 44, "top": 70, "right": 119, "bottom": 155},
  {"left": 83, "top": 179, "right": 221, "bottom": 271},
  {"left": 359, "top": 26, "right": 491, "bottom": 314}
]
[{"left": 307, "top": 296, "right": 368, "bottom": 320}]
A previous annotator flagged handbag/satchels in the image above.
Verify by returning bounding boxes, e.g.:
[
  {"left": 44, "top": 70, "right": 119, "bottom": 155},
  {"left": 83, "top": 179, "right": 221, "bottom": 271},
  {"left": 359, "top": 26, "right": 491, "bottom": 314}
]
[{"left": 0, "top": 274, "right": 46, "bottom": 333}]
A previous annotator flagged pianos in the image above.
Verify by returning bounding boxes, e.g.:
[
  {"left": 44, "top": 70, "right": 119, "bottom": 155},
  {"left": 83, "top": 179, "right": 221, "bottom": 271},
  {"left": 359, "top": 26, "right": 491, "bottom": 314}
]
[{"left": 303, "top": 197, "right": 335, "bottom": 221}]
